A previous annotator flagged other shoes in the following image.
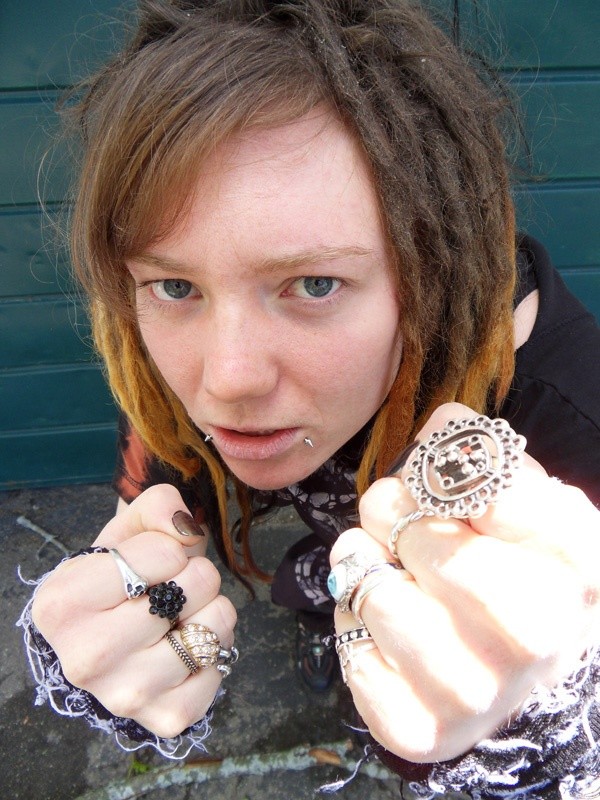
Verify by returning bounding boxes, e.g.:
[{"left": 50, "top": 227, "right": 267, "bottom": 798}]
[{"left": 295, "top": 622, "right": 338, "bottom": 695}]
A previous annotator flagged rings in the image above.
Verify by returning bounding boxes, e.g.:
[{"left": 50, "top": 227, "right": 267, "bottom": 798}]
[
  {"left": 110, "top": 548, "right": 149, "bottom": 600},
  {"left": 338, "top": 642, "right": 377, "bottom": 684},
  {"left": 351, "top": 568, "right": 415, "bottom": 625},
  {"left": 326, "top": 545, "right": 400, "bottom": 615},
  {"left": 175, "top": 622, "right": 221, "bottom": 669},
  {"left": 387, "top": 509, "right": 470, "bottom": 562},
  {"left": 164, "top": 631, "right": 198, "bottom": 675},
  {"left": 218, "top": 645, "right": 240, "bottom": 680},
  {"left": 333, "top": 627, "right": 373, "bottom": 654},
  {"left": 403, "top": 414, "right": 527, "bottom": 528},
  {"left": 146, "top": 580, "right": 187, "bottom": 629}
]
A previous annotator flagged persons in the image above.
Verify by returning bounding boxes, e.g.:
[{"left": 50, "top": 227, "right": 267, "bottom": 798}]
[{"left": 12, "top": 1, "right": 600, "bottom": 800}]
